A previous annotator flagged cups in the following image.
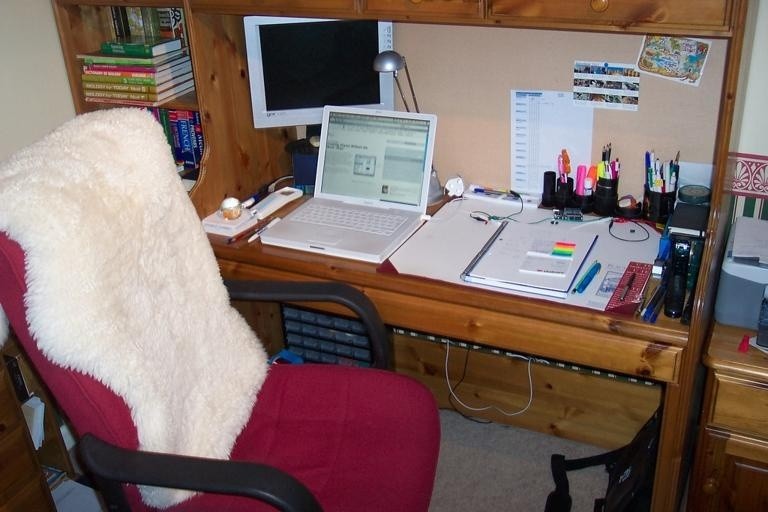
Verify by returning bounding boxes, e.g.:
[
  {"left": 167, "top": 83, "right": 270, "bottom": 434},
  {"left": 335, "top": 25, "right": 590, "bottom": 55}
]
[
  {"left": 641, "top": 184, "right": 679, "bottom": 224},
  {"left": 542, "top": 171, "right": 618, "bottom": 217}
]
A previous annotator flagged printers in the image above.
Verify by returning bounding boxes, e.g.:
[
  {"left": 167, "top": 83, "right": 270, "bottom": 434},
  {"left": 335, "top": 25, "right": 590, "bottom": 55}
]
[{"left": 714, "top": 216, "right": 768, "bottom": 330}]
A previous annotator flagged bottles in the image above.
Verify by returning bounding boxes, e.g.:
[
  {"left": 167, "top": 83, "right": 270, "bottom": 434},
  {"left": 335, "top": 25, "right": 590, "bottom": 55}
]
[{"left": 662, "top": 238, "right": 692, "bottom": 319}]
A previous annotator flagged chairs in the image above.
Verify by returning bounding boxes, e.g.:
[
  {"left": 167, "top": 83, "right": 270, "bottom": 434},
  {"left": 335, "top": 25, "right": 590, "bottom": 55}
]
[{"left": 0, "top": 106, "right": 442, "bottom": 511}]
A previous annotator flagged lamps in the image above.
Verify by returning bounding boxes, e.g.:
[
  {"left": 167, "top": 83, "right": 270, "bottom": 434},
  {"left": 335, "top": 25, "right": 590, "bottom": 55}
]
[{"left": 373, "top": 49, "right": 445, "bottom": 209}]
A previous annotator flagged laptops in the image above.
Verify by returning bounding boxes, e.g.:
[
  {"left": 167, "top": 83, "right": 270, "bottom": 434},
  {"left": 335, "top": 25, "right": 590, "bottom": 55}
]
[{"left": 260, "top": 105, "right": 438, "bottom": 265}]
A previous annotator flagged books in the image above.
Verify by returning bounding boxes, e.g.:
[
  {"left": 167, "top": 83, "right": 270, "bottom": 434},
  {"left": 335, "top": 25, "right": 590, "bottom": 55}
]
[
  {"left": 75, "top": 8, "right": 205, "bottom": 173},
  {"left": 459, "top": 220, "right": 599, "bottom": 300}
]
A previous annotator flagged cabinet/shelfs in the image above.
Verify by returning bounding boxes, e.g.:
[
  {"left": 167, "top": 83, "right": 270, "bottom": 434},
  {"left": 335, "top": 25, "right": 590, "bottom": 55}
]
[
  {"left": 681, "top": 316, "right": 768, "bottom": 512},
  {"left": 0, "top": 359, "right": 58, "bottom": 512}
]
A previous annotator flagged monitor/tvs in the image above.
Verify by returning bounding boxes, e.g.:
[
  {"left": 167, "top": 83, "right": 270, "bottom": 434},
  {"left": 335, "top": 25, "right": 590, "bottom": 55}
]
[{"left": 243, "top": 16, "right": 393, "bottom": 129}]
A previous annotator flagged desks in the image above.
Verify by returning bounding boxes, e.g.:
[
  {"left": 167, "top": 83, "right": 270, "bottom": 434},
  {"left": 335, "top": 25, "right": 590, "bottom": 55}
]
[{"left": 50, "top": 1, "right": 747, "bottom": 510}]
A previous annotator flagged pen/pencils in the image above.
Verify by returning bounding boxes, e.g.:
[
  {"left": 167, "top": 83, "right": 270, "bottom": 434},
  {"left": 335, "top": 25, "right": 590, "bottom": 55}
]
[
  {"left": 572, "top": 260, "right": 601, "bottom": 294},
  {"left": 620, "top": 273, "right": 636, "bottom": 301},
  {"left": 558, "top": 143, "right": 620, "bottom": 196},
  {"left": 645, "top": 286, "right": 668, "bottom": 323},
  {"left": 475, "top": 189, "right": 503, "bottom": 195},
  {"left": 228, "top": 215, "right": 280, "bottom": 244},
  {"left": 646, "top": 151, "right": 680, "bottom": 192}
]
